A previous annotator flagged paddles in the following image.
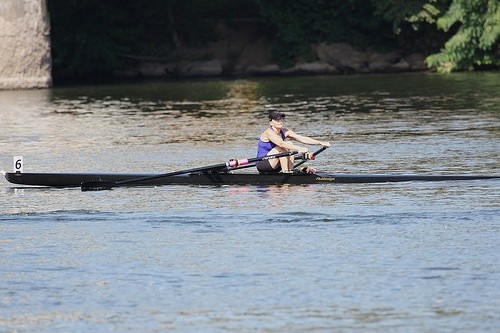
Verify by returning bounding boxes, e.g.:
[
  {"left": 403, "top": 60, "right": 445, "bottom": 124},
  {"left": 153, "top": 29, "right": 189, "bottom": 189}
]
[
  {"left": 80, "top": 150, "right": 299, "bottom": 191},
  {"left": 293, "top": 147, "right": 327, "bottom": 169}
]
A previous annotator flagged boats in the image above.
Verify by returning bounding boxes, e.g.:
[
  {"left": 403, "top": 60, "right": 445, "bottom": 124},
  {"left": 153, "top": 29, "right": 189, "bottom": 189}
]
[{"left": 4, "top": 155, "right": 500, "bottom": 187}]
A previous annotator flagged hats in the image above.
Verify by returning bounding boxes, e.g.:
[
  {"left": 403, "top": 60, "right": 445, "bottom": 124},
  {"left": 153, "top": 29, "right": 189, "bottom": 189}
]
[{"left": 269, "top": 112, "right": 285, "bottom": 121}]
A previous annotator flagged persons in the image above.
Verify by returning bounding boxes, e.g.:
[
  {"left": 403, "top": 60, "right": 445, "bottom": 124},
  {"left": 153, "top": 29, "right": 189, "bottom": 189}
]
[{"left": 256, "top": 111, "right": 331, "bottom": 174}]
